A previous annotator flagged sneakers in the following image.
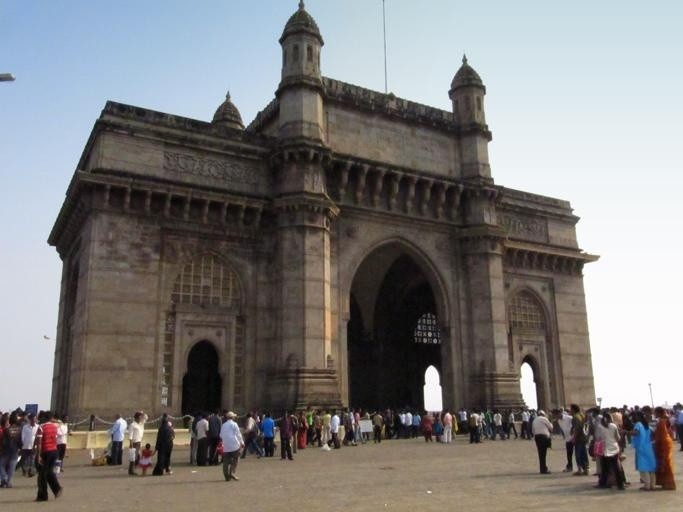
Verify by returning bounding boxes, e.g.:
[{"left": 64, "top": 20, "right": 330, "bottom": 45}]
[{"left": 562, "top": 468, "right": 589, "bottom": 475}]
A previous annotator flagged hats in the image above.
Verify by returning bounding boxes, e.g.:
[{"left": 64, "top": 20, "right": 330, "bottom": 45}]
[{"left": 225, "top": 412, "right": 236, "bottom": 419}]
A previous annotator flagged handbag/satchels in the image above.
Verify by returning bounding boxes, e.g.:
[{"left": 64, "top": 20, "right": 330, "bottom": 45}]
[
  {"left": 300, "top": 416, "right": 305, "bottom": 429},
  {"left": 128, "top": 441, "right": 135, "bottom": 463},
  {"left": 593, "top": 440, "right": 603, "bottom": 457},
  {"left": 588, "top": 440, "right": 594, "bottom": 456}
]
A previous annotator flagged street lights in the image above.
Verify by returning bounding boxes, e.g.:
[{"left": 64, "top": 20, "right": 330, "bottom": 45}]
[
  {"left": 647, "top": 382, "right": 654, "bottom": 407},
  {"left": 41, "top": 334, "right": 55, "bottom": 343},
  {"left": 0, "top": 72, "right": 16, "bottom": 83}
]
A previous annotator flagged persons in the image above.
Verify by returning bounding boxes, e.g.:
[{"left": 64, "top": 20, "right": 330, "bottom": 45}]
[
  {"left": 383, "top": 409, "right": 468, "bottom": 443},
  {"left": 278, "top": 404, "right": 382, "bottom": 460},
  {"left": 189, "top": 410, "right": 245, "bottom": 481},
  {"left": 0, "top": 406, "right": 68, "bottom": 501},
  {"left": 239, "top": 410, "right": 276, "bottom": 458},
  {"left": 106, "top": 410, "right": 175, "bottom": 475},
  {"left": 468, "top": 407, "right": 570, "bottom": 442},
  {"left": 532, "top": 405, "right": 683, "bottom": 491}
]
